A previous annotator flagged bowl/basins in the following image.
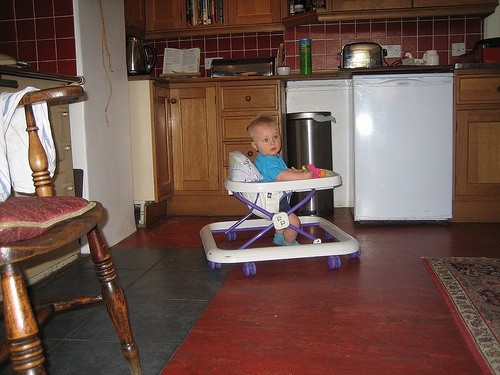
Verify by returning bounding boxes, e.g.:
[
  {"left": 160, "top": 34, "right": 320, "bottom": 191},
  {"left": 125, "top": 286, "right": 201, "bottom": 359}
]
[{"left": 276, "top": 66, "right": 290, "bottom": 76}]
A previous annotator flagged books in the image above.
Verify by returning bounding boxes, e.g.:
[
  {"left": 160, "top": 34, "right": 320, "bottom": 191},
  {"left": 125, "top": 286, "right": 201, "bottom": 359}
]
[
  {"left": 186, "top": 0, "right": 223, "bottom": 25},
  {"left": 163, "top": 48, "right": 201, "bottom": 74}
]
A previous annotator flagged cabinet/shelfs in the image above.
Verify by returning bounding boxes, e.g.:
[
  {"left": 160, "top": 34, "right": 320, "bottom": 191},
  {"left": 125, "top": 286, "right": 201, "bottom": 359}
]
[
  {"left": 124, "top": 0, "right": 281, "bottom": 38},
  {"left": 130, "top": 78, "right": 286, "bottom": 203},
  {"left": 282, "top": 1, "right": 499, "bottom": 26},
  {"left": 453, "top": 71, "right": 500, "bottom": 222}
]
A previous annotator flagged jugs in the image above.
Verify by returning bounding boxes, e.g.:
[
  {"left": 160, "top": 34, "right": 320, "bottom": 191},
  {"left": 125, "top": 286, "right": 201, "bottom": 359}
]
[{"left": 423, "top": 50, "right": 439, "bottom": 65}]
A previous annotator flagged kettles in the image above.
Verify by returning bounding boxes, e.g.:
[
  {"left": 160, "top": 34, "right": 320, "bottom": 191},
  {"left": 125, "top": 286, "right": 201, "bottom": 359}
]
[{"left": 126, "top": 36, "right": 156, "bottom": 76}]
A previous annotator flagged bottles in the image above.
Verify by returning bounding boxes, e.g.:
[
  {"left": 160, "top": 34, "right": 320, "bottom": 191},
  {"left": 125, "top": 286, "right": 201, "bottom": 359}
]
[
  {"left": 300, "top": 38, "right": 311, "bottom": 75},
  {"left": 289, "top": 3, "right": 295, "bottom": 15}
]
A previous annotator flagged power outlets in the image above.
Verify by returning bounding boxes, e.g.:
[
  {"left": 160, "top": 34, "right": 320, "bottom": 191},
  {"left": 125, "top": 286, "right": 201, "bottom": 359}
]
[{"left": 382, "top": 45, "right": 401, "bottom": 58}]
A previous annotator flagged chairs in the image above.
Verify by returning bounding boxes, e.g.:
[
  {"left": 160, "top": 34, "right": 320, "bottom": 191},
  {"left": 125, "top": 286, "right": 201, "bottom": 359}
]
[{"left": 0, "top": 85, "right": 142, "bottom": 375}]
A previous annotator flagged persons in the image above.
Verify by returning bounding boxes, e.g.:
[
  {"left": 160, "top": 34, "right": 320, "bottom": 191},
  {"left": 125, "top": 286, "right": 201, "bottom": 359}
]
[{"left": 248, "top": 115, "right": 314, "bottom": 247}]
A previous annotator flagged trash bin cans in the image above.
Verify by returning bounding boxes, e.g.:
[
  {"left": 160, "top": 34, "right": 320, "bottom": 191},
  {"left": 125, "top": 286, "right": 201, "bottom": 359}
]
[{"left": 287, "top": 112, "right": 333, "bottom": 216}]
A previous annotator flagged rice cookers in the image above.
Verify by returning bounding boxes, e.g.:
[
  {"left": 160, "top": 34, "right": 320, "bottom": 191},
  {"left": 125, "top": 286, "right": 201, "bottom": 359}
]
[{"left": 337, "top": 42, "right": 387, "bottom": 69}]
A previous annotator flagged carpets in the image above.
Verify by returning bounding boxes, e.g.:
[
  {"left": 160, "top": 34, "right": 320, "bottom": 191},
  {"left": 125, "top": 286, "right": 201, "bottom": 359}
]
[
  {"left": 1, "top": 246, "right": 234, "bottom": 375},
  {"left": 422, "top": 256, "right": 499, "bottom": 374}
]
